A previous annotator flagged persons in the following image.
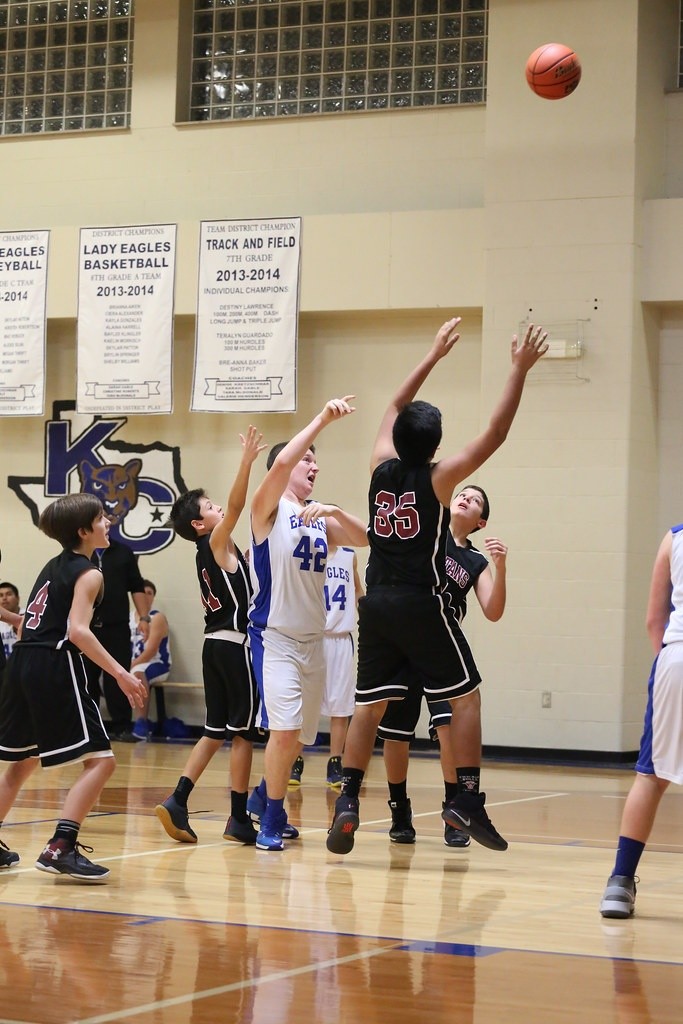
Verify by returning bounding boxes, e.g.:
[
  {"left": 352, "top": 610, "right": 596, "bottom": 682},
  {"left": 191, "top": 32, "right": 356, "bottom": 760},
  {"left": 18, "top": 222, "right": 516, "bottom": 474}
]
[
  {"left": 0, "top": 493, "right": 148, "bottom": 880},
  {"left": 325, "top": 317, "right": 549, "bottom": 855},
  {"left": 288, "top": 546, "right": 365, "bottom": 788},
  {"left": 154, "top": 425, "right": 268, "bottom": 845},
  {"left": 246, "top": 395, "right": 369, "bottom": 850},
  {"left": 0, "top": 582, "right": 26, "bottom": 665},
  {"left": 376, "top": 484, "right": 509, "bottom": 847},
  {"left": 86, "top": 539, "right": 151, "bottom": 741},
  {"left": 128, "top": 579, "right": 172, "bottom": 739},
  {"left": 600, "top": 522, "right": 683, "bottom": 919}
]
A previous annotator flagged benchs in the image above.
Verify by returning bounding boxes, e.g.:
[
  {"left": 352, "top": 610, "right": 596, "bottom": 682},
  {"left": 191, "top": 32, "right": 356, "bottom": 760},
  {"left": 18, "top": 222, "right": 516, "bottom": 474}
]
[{"left": 150, "top": 680, "right": 205, "bottom": 743}]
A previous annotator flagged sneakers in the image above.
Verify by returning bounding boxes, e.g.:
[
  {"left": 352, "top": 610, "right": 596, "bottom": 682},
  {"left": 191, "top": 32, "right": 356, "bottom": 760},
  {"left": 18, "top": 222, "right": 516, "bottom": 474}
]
[
  {"left": 258, "top": 809, "right": 287, "bottom": 851},
  {"left": 388, "top": 798, "right": 415, "bottom": 842},
  {"left": 601, "top": 873, "right": 640, "bottom": 917},
  {"left": 155, "top": 794, "right": 198, "bottom": 843},
  {"left": 444, "top": 798, "right": 471, "bottom": 847},
  {"left": 325, "top": 757, "right": 343, "bottom": 786},
  {"left": 288, "top": 755, "right": 305, "bottom": 784},
  {"left": 288, "top": 786, "right": 303, "bottom": 802},
  {"left": 36, "top": 837, "right": 111, "bottom": 880},
  {"left": 246, "top": 786, "right": 299, "bottom": 838},
  {"left": 444, "top": 788, "right": 507, "bottom": 851},
  {"left": 132, "top": 718, "right": 153, "bottom": 741},
  {"left": 600, "top": 919, "right": 634, "bottom": 953},
  {"left": 326, "top": 787, "right": 342, "bottom": 803},
  {"left": 225, "top": 813, "right": 260, "bottom": 844},
  {"left": 0, "top": 839, "right": 19, "bottom": 867},
  {"left": 326, "top": 794, "right": 360, "bottom": 854}
]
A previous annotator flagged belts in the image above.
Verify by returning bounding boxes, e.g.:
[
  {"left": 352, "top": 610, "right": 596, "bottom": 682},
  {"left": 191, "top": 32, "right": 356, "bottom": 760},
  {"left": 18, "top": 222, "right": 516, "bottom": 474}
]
[{"left": 202, "top": 628, "right": 250, "bottom": 647}]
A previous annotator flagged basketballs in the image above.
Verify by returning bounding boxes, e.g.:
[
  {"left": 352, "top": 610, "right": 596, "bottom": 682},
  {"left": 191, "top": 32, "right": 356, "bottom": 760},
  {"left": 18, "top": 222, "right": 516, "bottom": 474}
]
[{"left": 524, "top": 43, "right": 582, "bottom": 100}]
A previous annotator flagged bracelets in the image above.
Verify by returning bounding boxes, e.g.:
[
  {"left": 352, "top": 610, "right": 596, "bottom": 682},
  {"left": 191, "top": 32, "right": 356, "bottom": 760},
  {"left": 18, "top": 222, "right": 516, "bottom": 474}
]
[{"left": 140, "top": 616, "right": 151, "bottom": 623}]
